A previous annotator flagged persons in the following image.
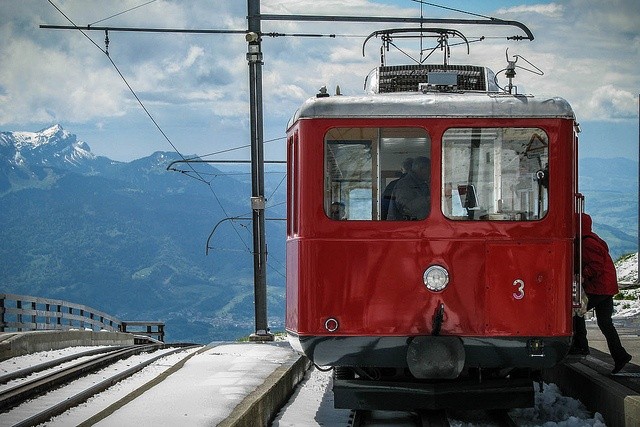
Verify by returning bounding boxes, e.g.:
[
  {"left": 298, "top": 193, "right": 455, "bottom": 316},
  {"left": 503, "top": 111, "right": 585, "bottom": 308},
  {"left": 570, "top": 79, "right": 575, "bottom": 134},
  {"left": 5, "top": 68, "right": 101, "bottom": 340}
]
[
  {"left": 330, "top": 203, "right": 347, "bottom": 221},
  {"left": 569, "top": 214, "right": 632, "bottom": 375},
  {"left": 381, "top": 159, "right": 413, "bottom": 220},
  {"left": 387, "top": 157, "right": 431, "bottom": 221}
]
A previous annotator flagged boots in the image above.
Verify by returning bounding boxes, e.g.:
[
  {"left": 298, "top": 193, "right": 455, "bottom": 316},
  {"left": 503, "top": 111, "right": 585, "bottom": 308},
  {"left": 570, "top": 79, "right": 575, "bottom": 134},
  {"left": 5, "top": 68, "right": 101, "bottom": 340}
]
[{"left": 609, "top": 349, "right": 632, "bottom": 375}]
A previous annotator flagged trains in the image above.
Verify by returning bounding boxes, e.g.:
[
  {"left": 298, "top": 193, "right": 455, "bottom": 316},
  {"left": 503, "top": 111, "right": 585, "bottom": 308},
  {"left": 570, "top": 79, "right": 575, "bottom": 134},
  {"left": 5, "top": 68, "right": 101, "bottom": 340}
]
[{"left": 286, "top": 28, "right": 591, "bottom": 418}]
